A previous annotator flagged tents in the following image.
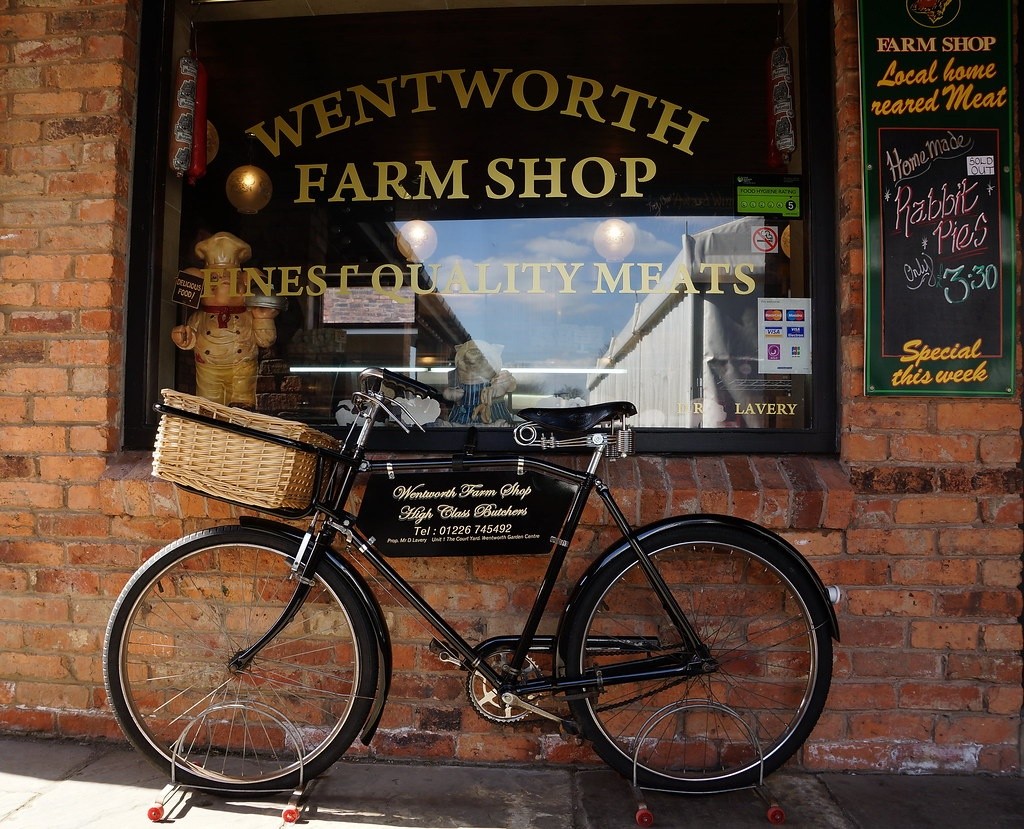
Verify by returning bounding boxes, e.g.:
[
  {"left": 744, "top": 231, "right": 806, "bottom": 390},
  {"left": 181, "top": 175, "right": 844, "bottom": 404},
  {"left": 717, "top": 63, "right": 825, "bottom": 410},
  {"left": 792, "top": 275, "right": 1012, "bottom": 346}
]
[{"left": 586, "top": 216, "right": 773, "bottom": 427}]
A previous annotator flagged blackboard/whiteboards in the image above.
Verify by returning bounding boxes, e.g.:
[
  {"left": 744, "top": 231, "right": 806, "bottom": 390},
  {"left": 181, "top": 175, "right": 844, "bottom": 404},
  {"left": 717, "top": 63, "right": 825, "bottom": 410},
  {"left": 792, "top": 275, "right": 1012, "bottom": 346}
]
[{"left": 858, "top": 0, "right": 1019, "bottom": 395}]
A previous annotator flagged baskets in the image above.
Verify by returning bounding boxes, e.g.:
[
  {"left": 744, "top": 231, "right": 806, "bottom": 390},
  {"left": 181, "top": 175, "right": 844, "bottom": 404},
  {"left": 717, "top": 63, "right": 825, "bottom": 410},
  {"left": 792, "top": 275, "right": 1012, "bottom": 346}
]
[{"left": 150, "top": 388, "right": 343, "bottom": 510}]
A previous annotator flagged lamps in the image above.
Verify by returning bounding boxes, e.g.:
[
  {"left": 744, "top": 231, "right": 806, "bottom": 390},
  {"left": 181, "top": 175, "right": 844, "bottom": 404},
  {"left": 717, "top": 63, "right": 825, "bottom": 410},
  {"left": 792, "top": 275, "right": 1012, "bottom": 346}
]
[
  {"left": 396, "top": 221, "right": 438, "bottom": 263},
  {"left": 593, "top": 219, "right": 633, "bottom": 263},
  {"left": 226, "top": 131, "right": 272, "bottom": 215}
]
